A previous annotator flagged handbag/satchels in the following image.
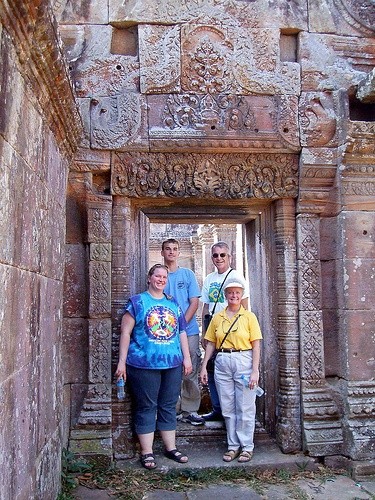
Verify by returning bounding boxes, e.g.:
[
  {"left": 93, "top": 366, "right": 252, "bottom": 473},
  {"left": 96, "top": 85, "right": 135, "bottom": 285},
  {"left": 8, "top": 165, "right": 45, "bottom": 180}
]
[
  {"left": 204, "top": 314, "right": 212, "bottom": 330},
  {"left": 207, "top": 351, "right": 218, "bottom": 373}
]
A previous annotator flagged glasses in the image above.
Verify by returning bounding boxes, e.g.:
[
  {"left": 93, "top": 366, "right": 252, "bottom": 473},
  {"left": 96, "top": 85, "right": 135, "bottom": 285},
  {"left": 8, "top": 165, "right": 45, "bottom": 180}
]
[
  {"left": 151, "top": 264, "right": 168, "bottom": 273},
  {"left": 212, "top": 253, "right": 226, "bottom": 258}
]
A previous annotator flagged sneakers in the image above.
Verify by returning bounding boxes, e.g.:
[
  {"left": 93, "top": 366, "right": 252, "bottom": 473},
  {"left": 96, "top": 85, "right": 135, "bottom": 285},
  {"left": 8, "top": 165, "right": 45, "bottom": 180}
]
[{"left": 181, "top": 411, "right": 205, "bottom": 426}]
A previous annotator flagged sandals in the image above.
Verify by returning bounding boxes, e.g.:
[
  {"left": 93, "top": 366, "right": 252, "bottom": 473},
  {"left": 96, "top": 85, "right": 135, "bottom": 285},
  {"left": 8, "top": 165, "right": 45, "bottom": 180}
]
[
  {"left": 223, "top": 450, "right": 255, "bottom": 462},
  {"left": 139, "top": 451, "right": 157, "bottom": 469},
  {"left": 164, "top": 448, "right": 189, "bottom": 463}
]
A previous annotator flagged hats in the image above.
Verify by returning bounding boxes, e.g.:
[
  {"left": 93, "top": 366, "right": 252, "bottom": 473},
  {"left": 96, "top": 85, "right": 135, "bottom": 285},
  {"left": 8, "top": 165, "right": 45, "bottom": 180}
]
[{"left": 223, "top": 278, "right": 245, "bottom": 295}]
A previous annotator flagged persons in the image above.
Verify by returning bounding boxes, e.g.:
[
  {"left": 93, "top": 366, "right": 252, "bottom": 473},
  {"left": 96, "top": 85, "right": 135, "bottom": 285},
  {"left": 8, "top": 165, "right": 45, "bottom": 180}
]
[
  {"left": 160, "top": 239, "right": 204, "bottom": 425},
  {"left": 200, "top": 241, "right": 250, "bottom": 423},
  {"left": 200, "top": 277, "right": 264, "bottom": 463},
  {"left": 115, "top": 264, "right": 192, "bottom": 469}
]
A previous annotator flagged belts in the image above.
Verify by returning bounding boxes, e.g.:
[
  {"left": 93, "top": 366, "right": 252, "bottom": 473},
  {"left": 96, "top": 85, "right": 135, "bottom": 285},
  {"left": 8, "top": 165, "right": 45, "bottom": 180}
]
[{"left": 215, "top": 349, "right": 252, "bottom": 353}]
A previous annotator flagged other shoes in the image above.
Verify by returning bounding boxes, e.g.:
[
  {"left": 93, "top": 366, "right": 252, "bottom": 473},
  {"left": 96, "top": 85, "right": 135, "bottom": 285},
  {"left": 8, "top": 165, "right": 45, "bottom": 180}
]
[{"left": 201, "top": 410, "right": 225, "bottom": 421}]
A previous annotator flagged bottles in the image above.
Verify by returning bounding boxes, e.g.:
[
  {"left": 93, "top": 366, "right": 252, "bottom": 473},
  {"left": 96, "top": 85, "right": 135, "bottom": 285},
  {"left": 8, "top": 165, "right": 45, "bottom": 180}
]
[
  {"left": 116, "top": 375, "right": 125, "bottom": 400},
  {"left": 241, "top": 375, "right": 264, "bottom": 398}
]
